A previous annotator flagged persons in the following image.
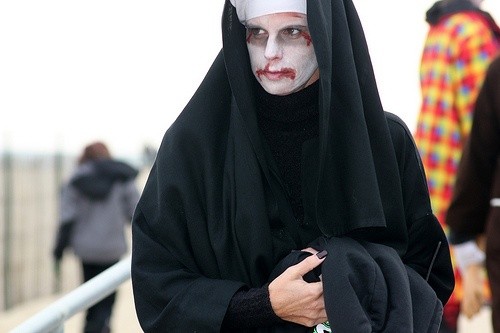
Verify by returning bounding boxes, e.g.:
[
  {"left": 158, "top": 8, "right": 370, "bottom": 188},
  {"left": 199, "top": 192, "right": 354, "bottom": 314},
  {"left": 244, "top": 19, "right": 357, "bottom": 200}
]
[
  {"left": 414, "top": 0, "right": 500, "bottom": 333},
  {"left": 130, "top": 0, "right": 455, "bottom": 333},
  {"left": 442, "top": 49, "right": 500, "bottom": 333},
  {"left": 49, "top": 141, "right": 139, "bottom": 333}
]
